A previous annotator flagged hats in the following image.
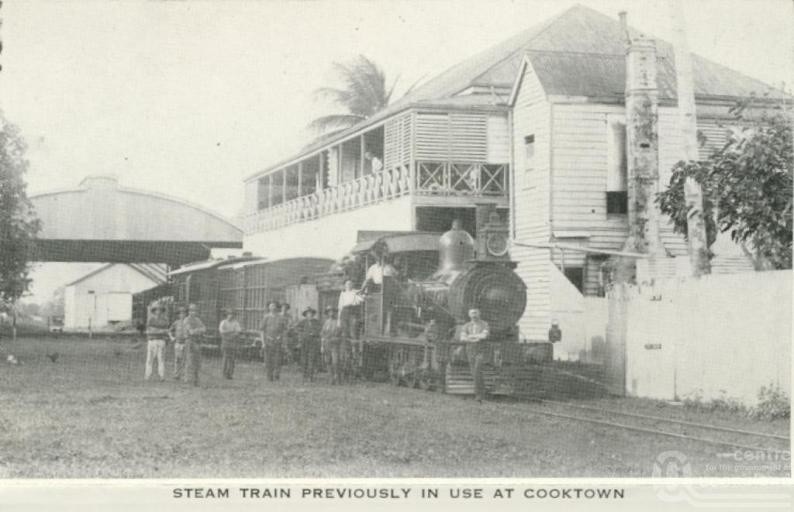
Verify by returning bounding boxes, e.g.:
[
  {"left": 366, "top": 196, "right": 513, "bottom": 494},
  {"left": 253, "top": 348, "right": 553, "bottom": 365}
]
[
  {"left": 176, "top": 307, "right": 187, "bottom": 314},
  {"left": 151, "top": 304, "right": 165, "bottom": 315},
  {"left": 303, "top": 306, "right": 316, "bottom": 317},
  {"left": 324, "top": 305, "right": 338, "bottom": 315},
  {"left": 279, "top": 303, "right": 290, "bottom": 311},
  {"left": 266, "top": 300, "right": 279, "bottom": 309},
  {"left": 224, "top": 308, "right": 236, "bottom": 314},
  {"left": 189, "top": 304, "right": 198, "bottom": 311}
]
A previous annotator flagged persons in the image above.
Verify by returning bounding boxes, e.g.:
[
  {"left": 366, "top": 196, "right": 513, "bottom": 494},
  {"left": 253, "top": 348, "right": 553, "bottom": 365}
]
[
  {"left": 143, "top": 301, "right": 172, "bottom": 382},
  {"left": 218, "top": 308, "right": 241, "bottom": 380},
  {"left": 364, "top": 152, "right": 382, "bottom": 173},
  {"left": 459, "top": 305, "right": 490, "bottom": 401},
  {"left": 182, "top": 303, "right": 207, "bottom": 388},
  {"left": 356, "top": 255, "right": 401, "bottom": 295},
  {"left": 336, "top": 278, "right": 361, "bottom": 337},
  {"left": 165, "top": 307, "right": 188, "bottom": 380},
  {"left": 262, "top": 294, "right": 342, "bottom": 385}
]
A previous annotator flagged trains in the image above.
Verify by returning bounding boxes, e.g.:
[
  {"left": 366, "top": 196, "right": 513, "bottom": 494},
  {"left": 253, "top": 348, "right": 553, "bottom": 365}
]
[{"left": 129, "top": 201, "right": 567, "bottom": 406}]
